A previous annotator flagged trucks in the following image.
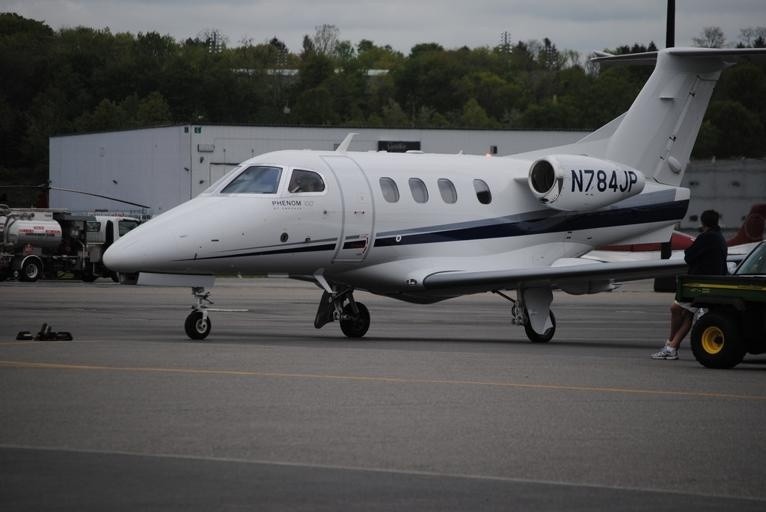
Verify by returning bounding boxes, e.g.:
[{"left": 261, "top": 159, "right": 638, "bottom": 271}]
[{"left": 0, "top": 207, "right": 142, "bottom": 282}]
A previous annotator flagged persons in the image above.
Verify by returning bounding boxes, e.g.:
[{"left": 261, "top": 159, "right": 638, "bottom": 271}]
[
  {"left": 487, "top": 144, "right": 497, "bottom": 158},
  {"left": 651, "top": 210, "right": 728, "bottom": 361}
]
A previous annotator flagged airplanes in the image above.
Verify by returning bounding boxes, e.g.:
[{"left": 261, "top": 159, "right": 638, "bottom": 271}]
[{"left": 102, "top": 46, "right": 766, "bottom": 344}]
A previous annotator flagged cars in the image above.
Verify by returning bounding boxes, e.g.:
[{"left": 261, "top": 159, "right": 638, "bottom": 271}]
[{"left": 677, "top": 238, "right": 766, "bottom": 367}]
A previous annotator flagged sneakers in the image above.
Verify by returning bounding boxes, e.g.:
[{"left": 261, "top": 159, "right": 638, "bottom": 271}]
[{"left": 650, "top": 339, "right": 680, "bottom": 360}]
